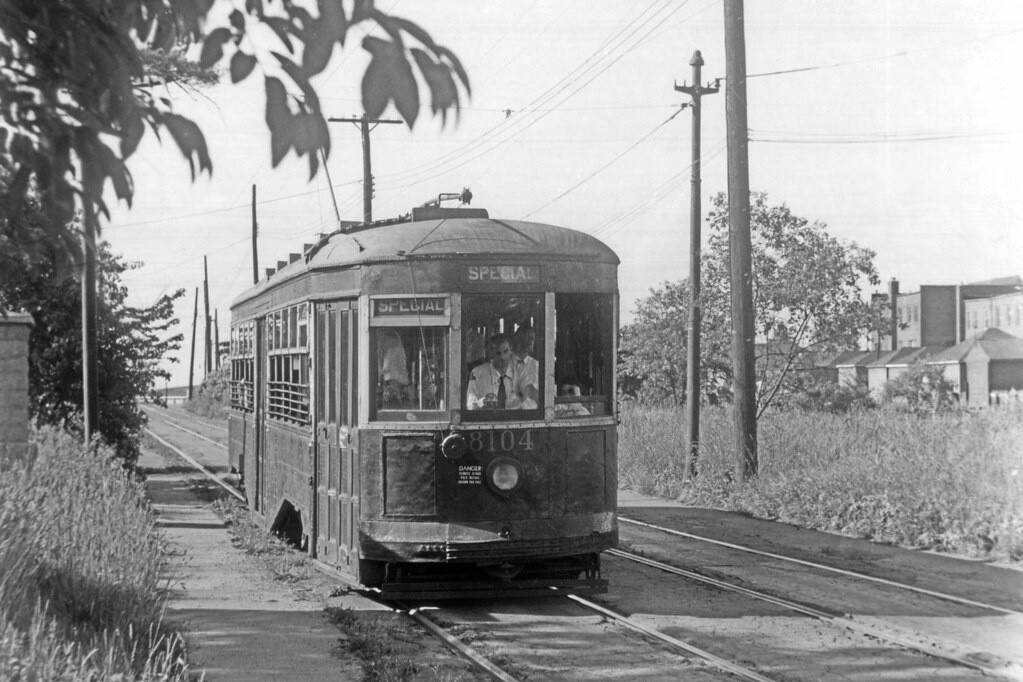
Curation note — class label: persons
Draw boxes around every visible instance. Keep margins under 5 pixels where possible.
[377,328,410,410]
[463,333,539,412]
[421,358,445,410]
[554,385,590,417]
[511,326,539,409]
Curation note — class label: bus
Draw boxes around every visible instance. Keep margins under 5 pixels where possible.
[227,207,620,603]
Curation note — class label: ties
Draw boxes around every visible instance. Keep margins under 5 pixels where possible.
[497,375,507,408]
